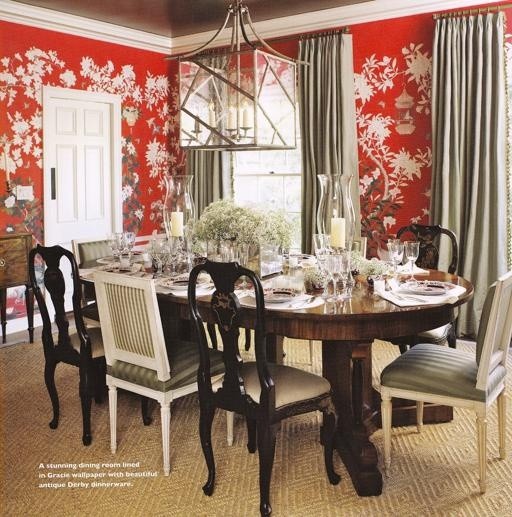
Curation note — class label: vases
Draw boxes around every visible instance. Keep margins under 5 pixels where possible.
[222,236,263,280]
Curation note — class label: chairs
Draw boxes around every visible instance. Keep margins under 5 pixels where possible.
[92,268,235,476]
[380,266,511,494]
[188,258,340,516]
[374,223,459,350]
[28,244,153,445]
[73,234,126,327]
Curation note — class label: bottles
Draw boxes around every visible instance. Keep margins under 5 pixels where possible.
[314,173,356,287]
[161,175,200,243]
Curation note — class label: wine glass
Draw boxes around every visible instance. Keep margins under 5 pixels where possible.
[100,230,280,279]
[284,236,422,307]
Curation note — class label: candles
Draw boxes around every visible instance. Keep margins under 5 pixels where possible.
[4,151,10,179]
[171,205,184,235]
[331,216,346,248]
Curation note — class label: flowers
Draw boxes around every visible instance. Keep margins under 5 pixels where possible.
[191,195,298,255]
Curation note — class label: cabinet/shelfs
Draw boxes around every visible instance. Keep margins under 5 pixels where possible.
[1,232,37,345]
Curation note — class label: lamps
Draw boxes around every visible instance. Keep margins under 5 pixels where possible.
[162,1,312,148]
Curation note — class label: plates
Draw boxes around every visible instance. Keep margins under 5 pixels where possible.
[400,279,452,296]
[96,256,118,267]
[249,288,298,305]
[160,277,207,290]
